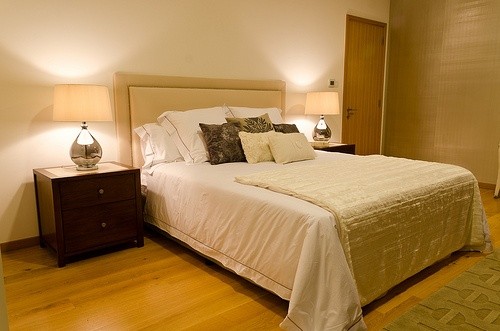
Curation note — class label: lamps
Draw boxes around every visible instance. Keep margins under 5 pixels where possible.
[52,83,114,169]
[305,91,341,142]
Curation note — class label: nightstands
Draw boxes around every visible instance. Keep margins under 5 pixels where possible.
[32,161,144,268]
[312,142,355,155]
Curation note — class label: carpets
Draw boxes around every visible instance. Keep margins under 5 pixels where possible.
[382,248,500,331]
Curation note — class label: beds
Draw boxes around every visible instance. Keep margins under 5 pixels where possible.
[114,71,493,331]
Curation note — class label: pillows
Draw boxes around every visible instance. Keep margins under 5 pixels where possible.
[270,132,315,162]
[159,106,234,164]
[199,123,248,165]
[238,131,277,163]
[228,106,284,123]
[134,123,182,165]
[225,114,274,132]
[272,123,300,133]
